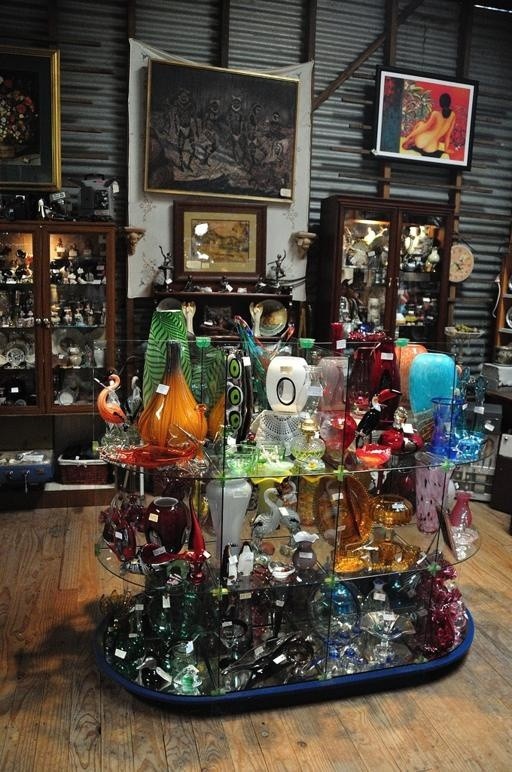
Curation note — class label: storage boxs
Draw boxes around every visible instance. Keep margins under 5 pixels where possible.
[0,448,110,485]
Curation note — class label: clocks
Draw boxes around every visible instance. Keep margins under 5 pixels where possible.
[448,245,473,282]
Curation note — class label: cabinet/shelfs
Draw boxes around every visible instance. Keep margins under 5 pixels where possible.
[319,197,451,344]
[0,217,117,413]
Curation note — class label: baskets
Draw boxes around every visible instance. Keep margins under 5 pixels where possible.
[56,443,115,486]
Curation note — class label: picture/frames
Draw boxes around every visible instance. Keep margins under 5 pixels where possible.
[141,57,302,205]
[169,198,267,280]
[367,63,479,173]
[0,40,66,193]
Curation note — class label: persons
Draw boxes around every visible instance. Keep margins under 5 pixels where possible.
[402,92,457,153]
[151,72,290,195]
[185,303,195,335]
[253,303,261,337]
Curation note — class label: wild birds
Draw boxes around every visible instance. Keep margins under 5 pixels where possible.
[125,375,143,423]
[276,481,303,546]
[249,486,284,543]
[97,374,126,433]
